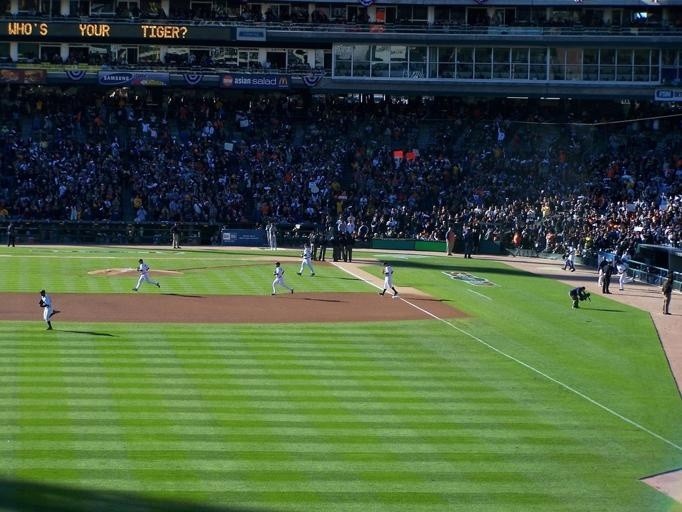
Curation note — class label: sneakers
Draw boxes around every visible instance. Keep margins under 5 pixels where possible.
[297,273,301,275]
[53,309,60,313]
[310,273,315,277]
[156,282,160,288]
[132,288,138,291]
[379,293,384,296]
[311,258,352,262]
[272,293,277,295]
[46,327,52,330]
[394,292,398,296]
[291,288,293,294]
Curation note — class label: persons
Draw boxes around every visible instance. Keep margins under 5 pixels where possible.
[601,261,613,294]
[660,273,674,315]
[378,262,398,296]
[569,287,588,308]
[0,2,682,291]
[37,289,62,331]
[270,261,293,296]
